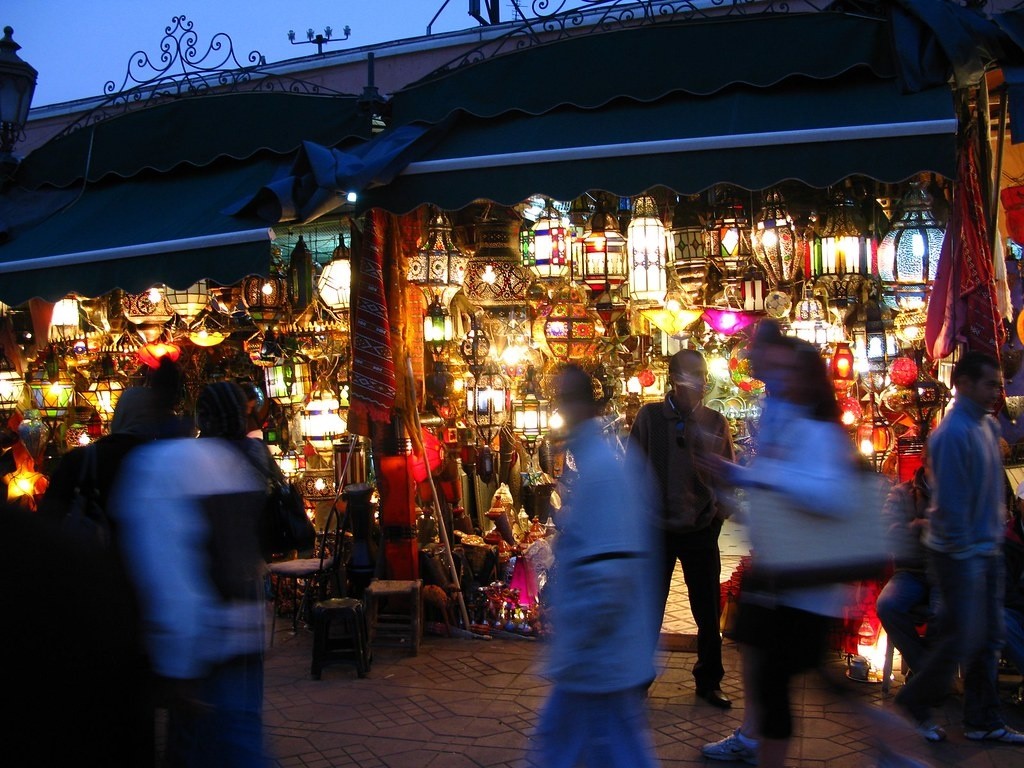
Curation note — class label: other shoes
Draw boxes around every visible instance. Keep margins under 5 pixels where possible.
[962,723,1024,745]
[694,686,732,710]
[761,720,792,739]
[919,715,951,743]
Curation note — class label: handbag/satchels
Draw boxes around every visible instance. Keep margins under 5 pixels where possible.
[746,474,898,592]
[201,485,319,599]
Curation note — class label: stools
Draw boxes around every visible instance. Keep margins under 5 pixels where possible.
[366,576,424,655]
[312,596,372,679]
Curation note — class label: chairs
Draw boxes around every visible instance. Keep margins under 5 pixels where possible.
[266,493,347,648]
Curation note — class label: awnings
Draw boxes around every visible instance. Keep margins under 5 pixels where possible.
[223,7,969,224]
[1,91,346,308]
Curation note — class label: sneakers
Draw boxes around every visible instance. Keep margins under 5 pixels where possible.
[700,727,762,766]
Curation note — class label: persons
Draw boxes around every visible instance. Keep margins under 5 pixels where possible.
[876,449,1015,703]
[524,364,660,768]
[627,349,734,710]
[0,353,283,768]
[696,317,863,764]
[894,351,1024,744]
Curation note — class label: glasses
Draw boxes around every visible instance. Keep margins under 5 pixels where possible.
[676,419,687,450]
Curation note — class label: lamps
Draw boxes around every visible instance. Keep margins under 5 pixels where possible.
[0,171,955,524]
